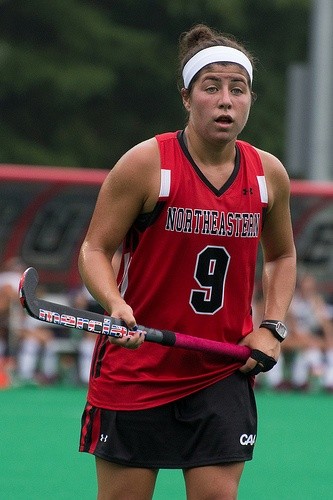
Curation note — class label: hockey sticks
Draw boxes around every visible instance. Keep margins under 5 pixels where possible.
[18,267,252,362]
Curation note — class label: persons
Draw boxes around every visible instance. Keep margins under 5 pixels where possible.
[77,26,297,499]
[248,270,333,392]
[0,254,108,388]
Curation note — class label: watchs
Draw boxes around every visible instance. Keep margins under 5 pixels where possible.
[260,318,288,343]
